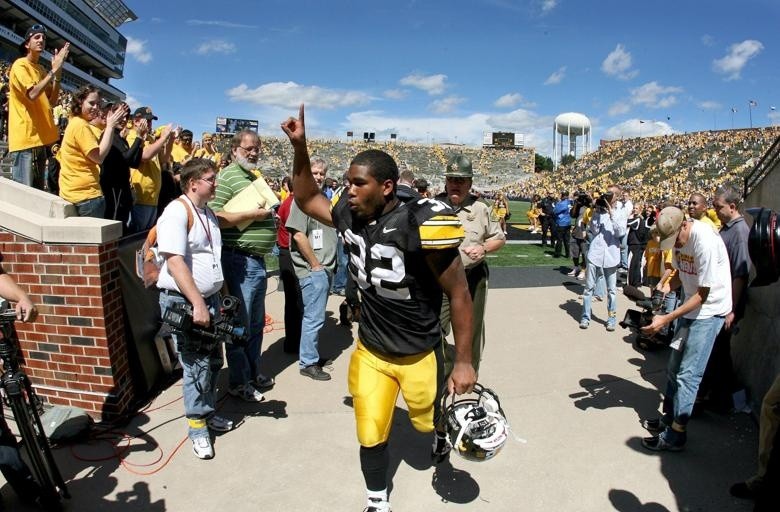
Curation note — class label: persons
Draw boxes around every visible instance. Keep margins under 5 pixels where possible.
[156,157,235,460]
[529,126,780,460]
[212,132,537,381]
[0,24,225,360]
[725,378,779,499]
[275,102,474,512]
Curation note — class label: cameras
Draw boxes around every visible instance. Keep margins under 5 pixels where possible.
[745,206,780,288]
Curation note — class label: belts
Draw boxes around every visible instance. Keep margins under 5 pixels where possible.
[221,246,264,262]
[159,288,185,298]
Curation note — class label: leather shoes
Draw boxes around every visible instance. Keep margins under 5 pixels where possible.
[300,364,331,381]
[316,357,332,366]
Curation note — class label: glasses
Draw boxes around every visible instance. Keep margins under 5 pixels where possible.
[25,24,48,39]
[197,177,216,184]
[238,145,260,153]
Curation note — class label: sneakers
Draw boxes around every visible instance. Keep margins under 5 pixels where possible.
[191,435,214,459]
[227,382,264,402]
[248,373,275,387]
[283,339,300,355]
[617,266,629,273]
[642,414,674,431]
[527,225,586,280]
[641,426,686,453]
[361,497,389,512]
[605,317,616,331]
[207,415,233,432]
[578,317,590,329]
[430,431,452,462]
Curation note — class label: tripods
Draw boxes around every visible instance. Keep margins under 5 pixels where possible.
[1,369,72,510]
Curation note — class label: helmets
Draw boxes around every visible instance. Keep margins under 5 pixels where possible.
[442,395,509,463]
[442,155,473,178]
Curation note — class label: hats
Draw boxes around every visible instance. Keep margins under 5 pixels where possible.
[655,206,684,251]
[99,96,115,109]
[560,190,570,196]
[133,106,158,121]
[19,24,48,53]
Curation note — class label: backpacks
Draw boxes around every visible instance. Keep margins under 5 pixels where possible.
[135,196,194,288]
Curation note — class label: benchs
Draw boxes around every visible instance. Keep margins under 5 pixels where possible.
[0,141,14,180]
